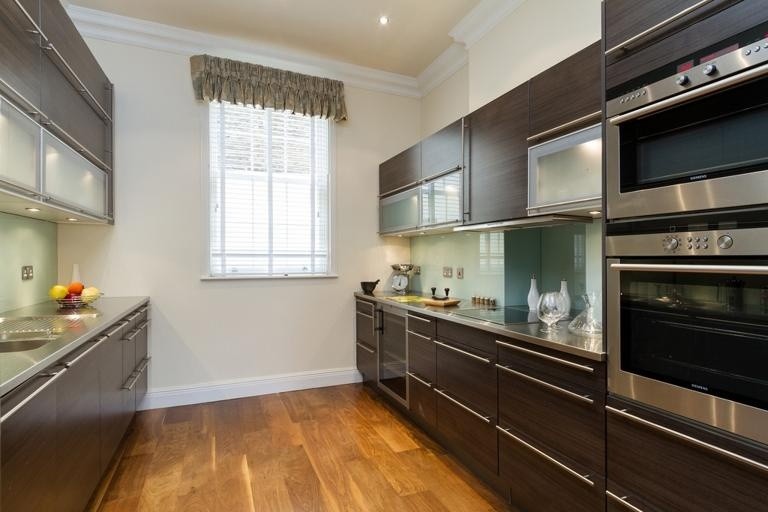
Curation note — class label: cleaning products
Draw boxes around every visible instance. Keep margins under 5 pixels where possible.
[527,274,540,313]
[528,311,539,332]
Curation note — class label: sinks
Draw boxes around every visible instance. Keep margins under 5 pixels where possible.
[0,338,56,352]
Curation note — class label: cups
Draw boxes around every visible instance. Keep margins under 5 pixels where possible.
[360,282,377,293]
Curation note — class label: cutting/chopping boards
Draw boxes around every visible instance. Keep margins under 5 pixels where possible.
[422,299,460,308]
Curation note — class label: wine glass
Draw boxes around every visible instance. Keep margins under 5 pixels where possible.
[535,292,568,333]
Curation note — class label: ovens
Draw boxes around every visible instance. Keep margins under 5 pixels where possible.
[603,36,767,447]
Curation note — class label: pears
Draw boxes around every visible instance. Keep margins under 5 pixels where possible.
[80,287,104,304]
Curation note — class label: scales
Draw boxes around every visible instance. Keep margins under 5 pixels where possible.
[390,264,413,296]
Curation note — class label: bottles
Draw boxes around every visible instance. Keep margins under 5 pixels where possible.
[568,294,602,338]
[70,263,82,284]
[526,272,539,311]
[471,296,496,306]
[527,311,541,336]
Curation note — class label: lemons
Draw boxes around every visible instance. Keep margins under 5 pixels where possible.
[48,286,68,299]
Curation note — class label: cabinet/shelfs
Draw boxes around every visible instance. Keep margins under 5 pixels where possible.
[60,336,104,512]
[354,293,379,392]
[120,309,141,441]
[434,316,496,489]
[136,302,153,409]
[604,389,767,511]
[524,39,602,221]
[0,346,69,512]
[460,78,529,230]
[40,0,117,226]
[97,321,128,470]
[0,1,39,221]
[598,0,766,102]
[496,331,605,512]
[406,309,434,438]
[377,110,461,240]
